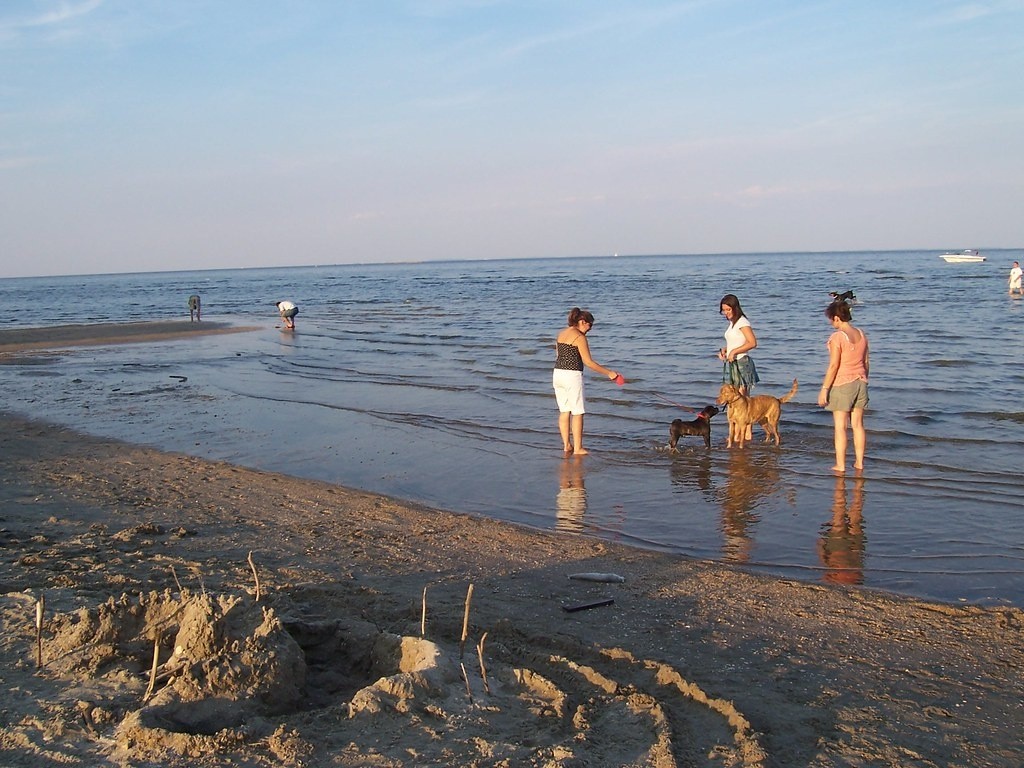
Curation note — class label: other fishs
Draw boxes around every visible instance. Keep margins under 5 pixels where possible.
[568,572,625,584]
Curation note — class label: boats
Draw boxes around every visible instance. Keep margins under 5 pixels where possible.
[938,248,986,265]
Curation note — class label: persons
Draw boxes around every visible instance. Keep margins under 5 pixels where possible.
[553,307,621,455]
[276,301,299,328]
[1008,261,1024,295]
[718,294,760,441]
[818,301,869,471]
[188,295,201,322]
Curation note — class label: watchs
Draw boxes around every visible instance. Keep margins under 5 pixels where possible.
[822,386,829,390]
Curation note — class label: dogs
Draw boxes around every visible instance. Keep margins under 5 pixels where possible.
[716,378,798,450]
[669,405,719,448]
